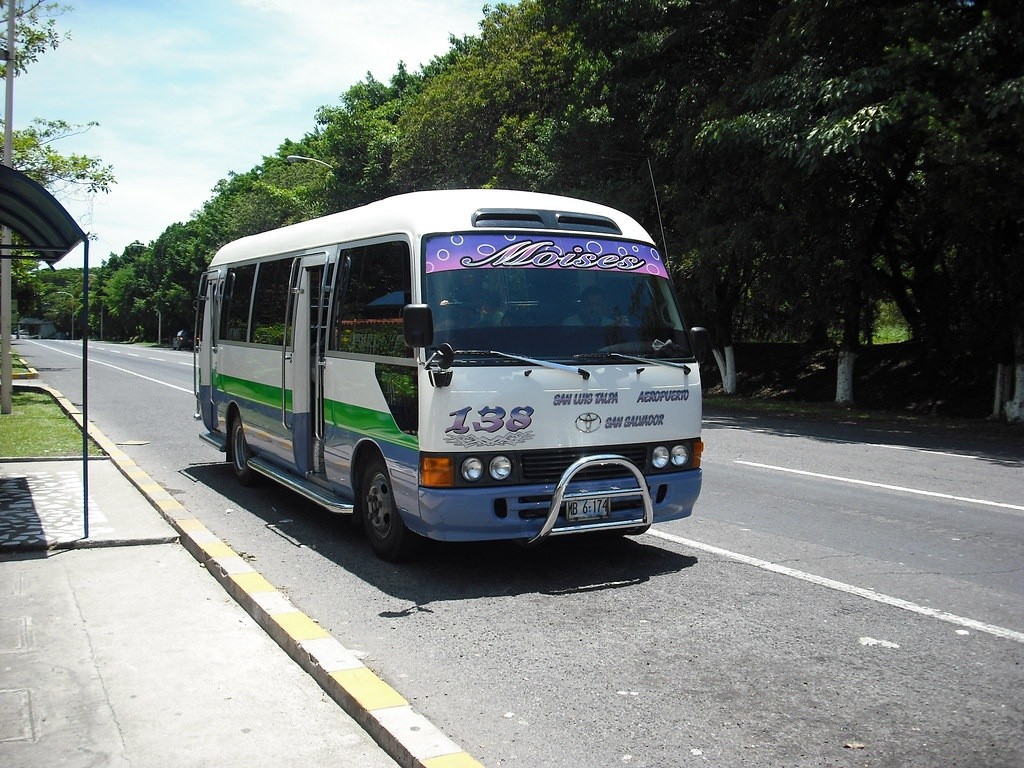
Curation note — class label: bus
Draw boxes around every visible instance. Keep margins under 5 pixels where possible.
[193,189,709,565]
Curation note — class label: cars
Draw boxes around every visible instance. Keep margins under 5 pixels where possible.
[173,329,199,351]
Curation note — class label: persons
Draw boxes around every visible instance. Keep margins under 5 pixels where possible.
[561,287,633,354]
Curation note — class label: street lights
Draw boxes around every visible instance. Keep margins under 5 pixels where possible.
[58,291,74,340]
